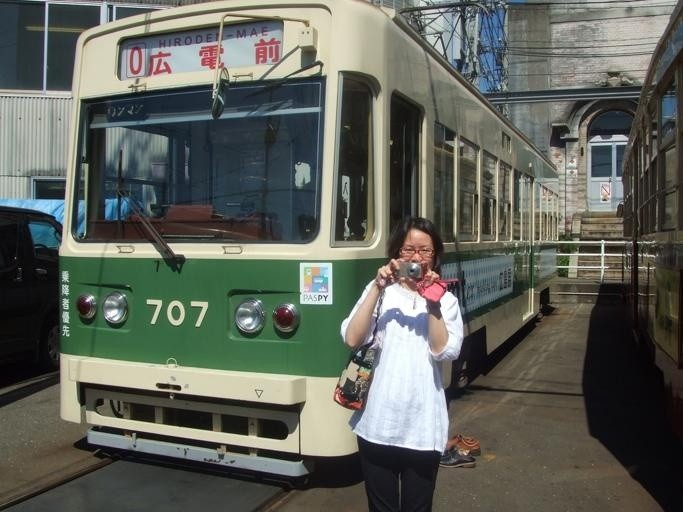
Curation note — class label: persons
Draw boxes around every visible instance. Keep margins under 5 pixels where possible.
[332,214,463,510]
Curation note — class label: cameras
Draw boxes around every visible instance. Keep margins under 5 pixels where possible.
[394,261,423,280]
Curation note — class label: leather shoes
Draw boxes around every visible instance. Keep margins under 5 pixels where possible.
[439,434,480,467]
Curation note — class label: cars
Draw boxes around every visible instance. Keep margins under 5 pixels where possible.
[0,205,63,375]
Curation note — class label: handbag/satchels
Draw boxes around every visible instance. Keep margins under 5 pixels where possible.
[334,338,378,410]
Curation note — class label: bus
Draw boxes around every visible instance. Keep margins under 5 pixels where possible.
[53,0,560,481]
[619,3,679,409]
